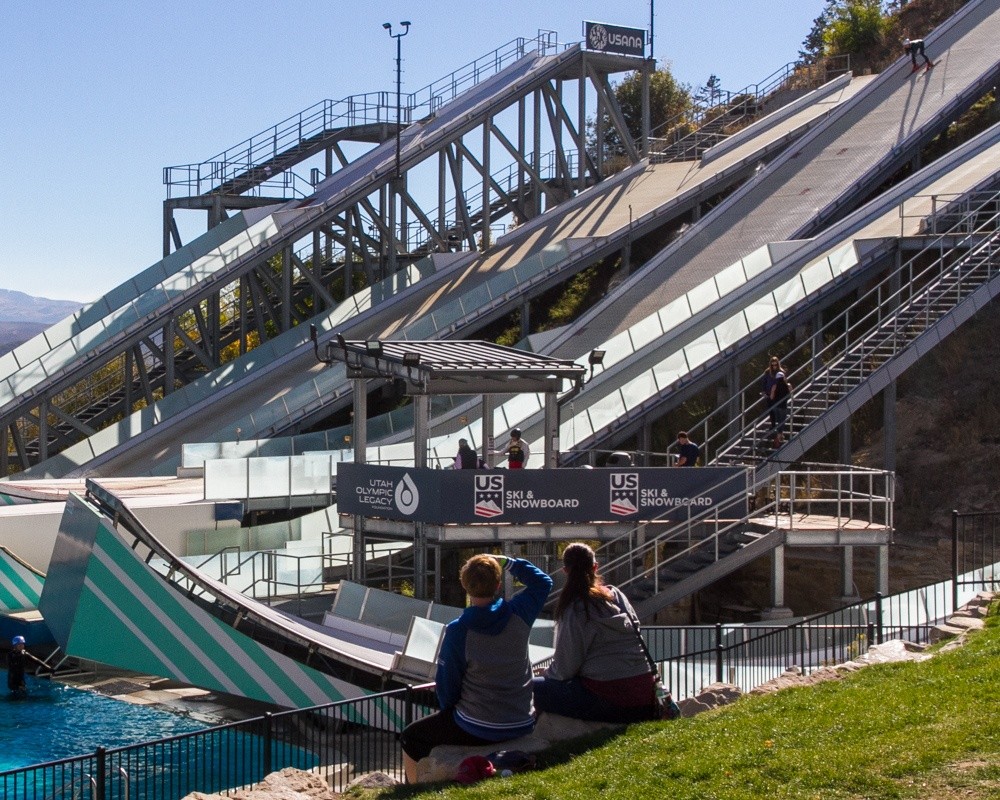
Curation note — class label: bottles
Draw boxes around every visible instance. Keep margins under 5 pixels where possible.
[656,681,670,708]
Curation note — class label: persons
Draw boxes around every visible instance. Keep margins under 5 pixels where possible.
[401,553,554,785]
[7,636,25,696]
[760,357,792,447]
[534,542,659,723]
[673,432,698,467]
[454,439,479,470]
[903,39,934,72]
[498,428,530,469]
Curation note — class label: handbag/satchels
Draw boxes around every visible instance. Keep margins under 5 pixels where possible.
[650,671,681,719]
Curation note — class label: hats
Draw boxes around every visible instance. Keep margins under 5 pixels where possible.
[775,371,784,379]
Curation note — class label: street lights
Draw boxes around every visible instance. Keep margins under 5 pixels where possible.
[381,19,414,180]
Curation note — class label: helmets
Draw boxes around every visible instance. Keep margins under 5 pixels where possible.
[510,428,521,440]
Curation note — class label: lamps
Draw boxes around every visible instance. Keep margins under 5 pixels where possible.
[582,351,605,385]
[336,332,362,371]
[403,353,424,388]
[365,339,384,374]
[310,323,333,364]
